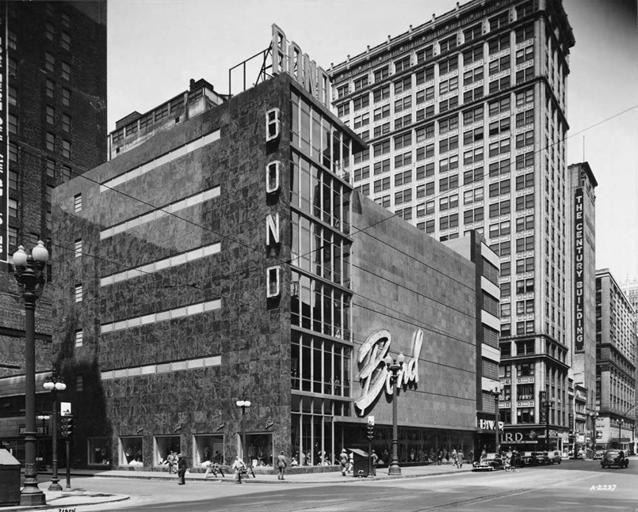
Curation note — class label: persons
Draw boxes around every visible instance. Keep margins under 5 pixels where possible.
[370,450,378,476]
[166,449,354,485]
[437,447,522,472]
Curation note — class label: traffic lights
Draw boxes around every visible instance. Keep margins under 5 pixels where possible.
[65,416,74,440]
[59,414,65,440]
[371,427,375,440]
[366,427,370,440]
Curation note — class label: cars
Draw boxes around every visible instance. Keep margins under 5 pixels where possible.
[470,446,630,472]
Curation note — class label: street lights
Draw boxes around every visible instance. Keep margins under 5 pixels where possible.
[540,396,556,448]
[237,385,250,470]
[42,371,66,492]
[588,410,600,452]
[616,418,624,449]
[378,350,407,476]
[488,381,505,453]
[12,240,49,505]
